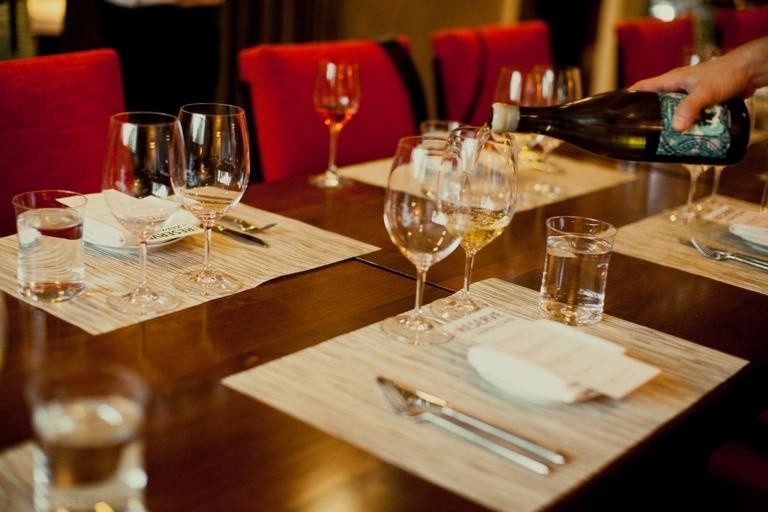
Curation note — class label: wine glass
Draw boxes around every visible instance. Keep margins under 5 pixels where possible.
[432,127,518,323]
[169,103,251,297]
[306,61,361,190]
[496,67,582,204]
[102,111,187,317]
[663,164,727,225]
[379,136,471,346]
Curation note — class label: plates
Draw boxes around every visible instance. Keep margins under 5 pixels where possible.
[465,319,626,402]
[73,198,201,254]
[728,224,768,251]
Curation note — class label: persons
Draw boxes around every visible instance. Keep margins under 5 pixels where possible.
[627,34,768,132]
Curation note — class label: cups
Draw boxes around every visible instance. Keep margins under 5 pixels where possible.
[537,216,616,326]
[684,44,721,67]
[27,360,152,512]
[12,190,87,303]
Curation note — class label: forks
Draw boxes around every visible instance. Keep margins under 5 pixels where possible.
[377,377,549,476]
[691,237,768,272]
[222,212,277,233]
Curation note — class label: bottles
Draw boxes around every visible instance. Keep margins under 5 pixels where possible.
[488,90,750,166]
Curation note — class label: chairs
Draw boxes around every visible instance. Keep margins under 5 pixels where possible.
[238,35,431,182]
[715,7,768,44]
[428,21,554,133]
[616,12,698,89]
[0,48,135,236]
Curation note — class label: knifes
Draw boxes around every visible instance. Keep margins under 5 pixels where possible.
[391,380,565,465]
[210,222,269,252]
[678,237,768,268]
